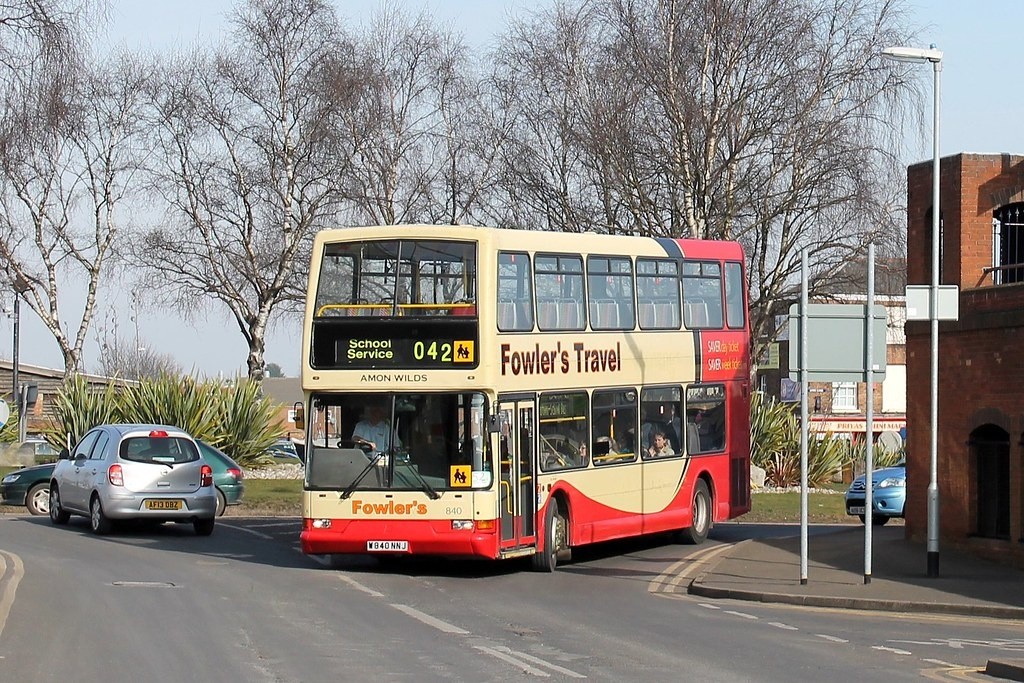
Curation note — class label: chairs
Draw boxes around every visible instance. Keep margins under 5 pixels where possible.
[345,298,735,331]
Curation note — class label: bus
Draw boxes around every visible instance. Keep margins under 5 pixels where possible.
[292,223,754,572]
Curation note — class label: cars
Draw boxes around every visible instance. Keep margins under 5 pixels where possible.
[49,423,217,536]
[845,456,907,525]
[266,441,295,457]
[0,436,245,515]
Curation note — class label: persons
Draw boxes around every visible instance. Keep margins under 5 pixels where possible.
[351,409,402,459]
[597,436,622,462]
[648,430,674,457]
[578,439,586,457]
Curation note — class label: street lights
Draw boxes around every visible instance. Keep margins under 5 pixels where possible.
[11,274,25,409]
[883,47,944,578]
